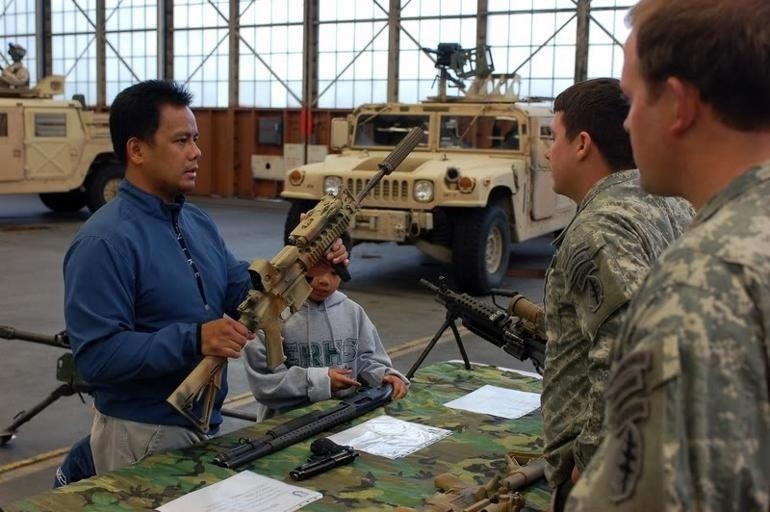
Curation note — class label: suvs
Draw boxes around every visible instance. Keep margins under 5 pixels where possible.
[280,44,579,298]
[0,74,127,214]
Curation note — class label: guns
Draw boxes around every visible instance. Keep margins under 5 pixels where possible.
[213,382,393,468]
[406,275,548,379]
[289,437,360,481]
[424,449,544,512]
[165,126,425,434]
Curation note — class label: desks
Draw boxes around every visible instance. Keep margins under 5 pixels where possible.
[0,356,555,511]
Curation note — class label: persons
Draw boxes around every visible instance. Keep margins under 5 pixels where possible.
[239,198,412,425]
[558,1,770,512]
[60,77,350,480]
[538,77,697,512]
[1,44,30,88]
